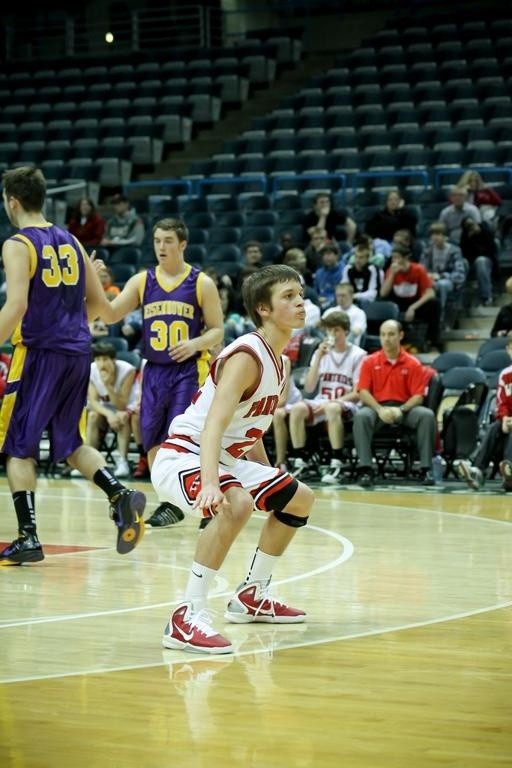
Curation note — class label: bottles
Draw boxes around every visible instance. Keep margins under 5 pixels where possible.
[326,333,336,356]
[430,453,444,484]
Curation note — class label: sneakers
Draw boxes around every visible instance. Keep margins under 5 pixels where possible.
[452,459,484,490]
[274,457,373,487]
[224,579,308,624]
[53,465,84,479]
[144,502,185,529]
[0,528,44,566]
[133,457,149,477]
[497,460,512,492]
[109,487,146,554]
[162,601,235,654]
[418,468,435,485]
[114,461,130,478]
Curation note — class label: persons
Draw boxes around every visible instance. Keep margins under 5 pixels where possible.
[0,164,146,568]
[150,265,312,656]
[95,215,228,529]
[2,167,512,492]
